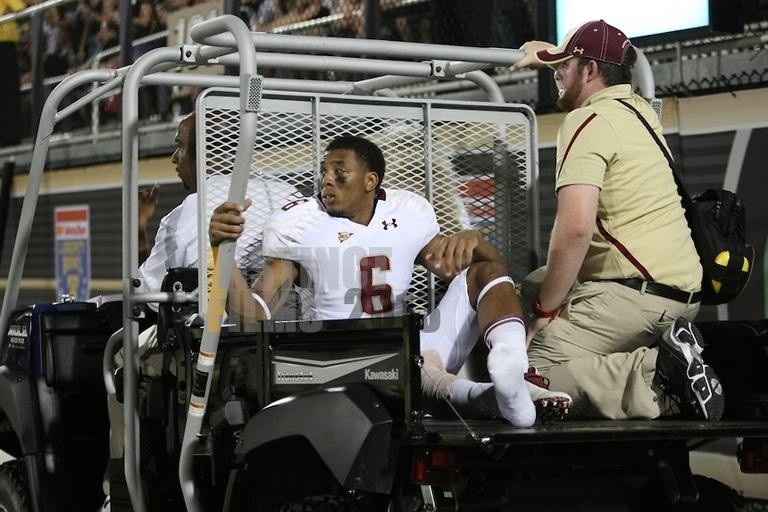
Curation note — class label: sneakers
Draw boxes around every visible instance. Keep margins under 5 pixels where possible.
[522,366,572,421]
[652,317,726,423]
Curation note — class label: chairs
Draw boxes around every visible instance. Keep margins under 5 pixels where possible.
[115,268,198,404]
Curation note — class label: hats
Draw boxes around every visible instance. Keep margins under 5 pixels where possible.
[536,20,635,69]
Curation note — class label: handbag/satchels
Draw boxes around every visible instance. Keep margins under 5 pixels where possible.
[688,188,755,307]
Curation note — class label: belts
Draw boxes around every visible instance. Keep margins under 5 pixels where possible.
[614,278,701,306]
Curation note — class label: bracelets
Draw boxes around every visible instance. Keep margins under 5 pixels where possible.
[532,295,560,319]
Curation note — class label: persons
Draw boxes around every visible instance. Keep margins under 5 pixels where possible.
[207,131,575,429]
[508,17,729,421]
[96,108,305,510]
[0,1,535,146]
